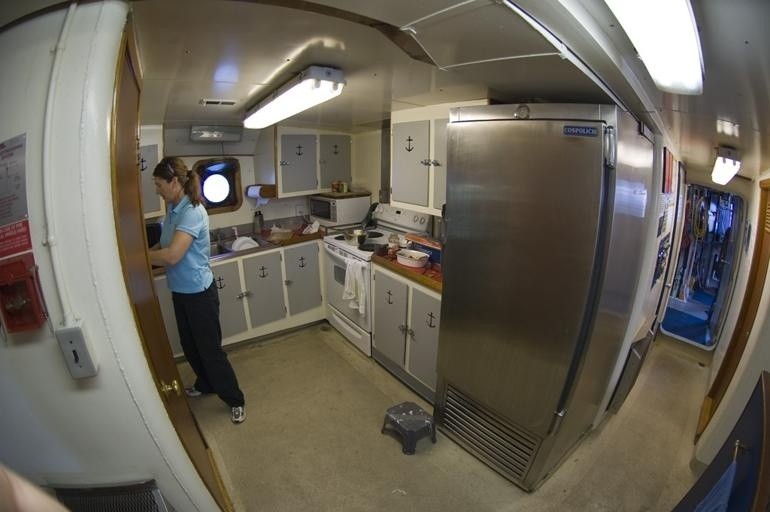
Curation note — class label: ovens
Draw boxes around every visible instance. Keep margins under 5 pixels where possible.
[323,241,371,334]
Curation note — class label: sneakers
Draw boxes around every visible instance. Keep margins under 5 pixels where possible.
[185,387,202,397]
[231,406,246,423]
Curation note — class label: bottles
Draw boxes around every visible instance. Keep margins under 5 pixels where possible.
[254,211,264,234]
[331,181,349,193]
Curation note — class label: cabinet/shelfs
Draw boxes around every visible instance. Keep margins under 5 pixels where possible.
[139,125,166,220]
[372,269,441,407]
[255,125,352,201]
[390,99,489,218]
[153,240,322,363]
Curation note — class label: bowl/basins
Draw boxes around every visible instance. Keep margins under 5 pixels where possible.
[395,248,430,268]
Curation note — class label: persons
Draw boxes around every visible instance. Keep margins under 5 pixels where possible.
[145,154,249,427]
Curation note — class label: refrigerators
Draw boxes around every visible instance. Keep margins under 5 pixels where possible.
[431,104,656,494]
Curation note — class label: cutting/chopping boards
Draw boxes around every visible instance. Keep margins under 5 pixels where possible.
[266,232,295,245]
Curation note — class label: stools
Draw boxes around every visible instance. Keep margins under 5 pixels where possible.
[382,402,437,455]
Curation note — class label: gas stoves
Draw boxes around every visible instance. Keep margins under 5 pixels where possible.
[323,226,408,262]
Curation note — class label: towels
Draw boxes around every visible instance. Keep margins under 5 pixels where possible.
[341,258,366,313]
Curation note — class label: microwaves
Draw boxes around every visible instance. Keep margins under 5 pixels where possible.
[308,196,371,228]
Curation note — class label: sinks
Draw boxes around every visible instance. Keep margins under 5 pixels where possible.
[223,236,269,251]
[210,241,232,260]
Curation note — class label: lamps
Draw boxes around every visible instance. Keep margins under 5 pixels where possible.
[711,146,742,186]
[242,66,348,130]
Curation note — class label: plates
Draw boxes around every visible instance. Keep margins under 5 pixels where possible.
[231,236,259,251]
